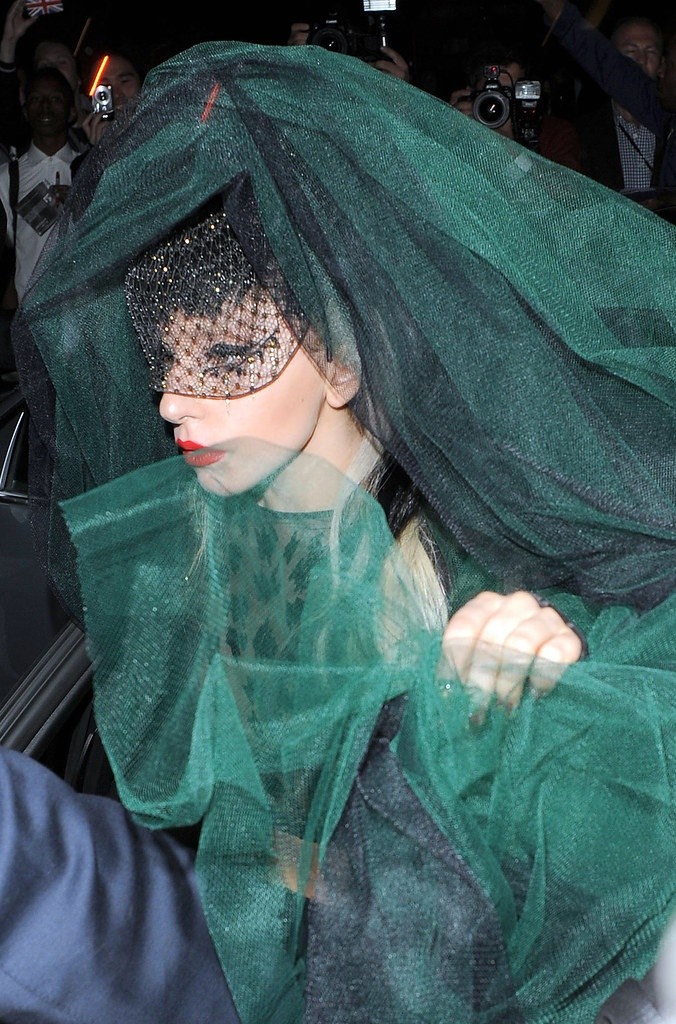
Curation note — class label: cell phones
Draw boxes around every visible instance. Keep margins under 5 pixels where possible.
[25,0,64,17]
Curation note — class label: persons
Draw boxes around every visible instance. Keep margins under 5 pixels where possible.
[0,0,676,1024]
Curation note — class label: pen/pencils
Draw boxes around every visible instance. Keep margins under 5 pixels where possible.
[55,171,60,208]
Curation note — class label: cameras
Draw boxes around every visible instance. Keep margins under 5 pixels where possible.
[463,63,547,152]
[307,0,397,66]
[92,84,115,123]
